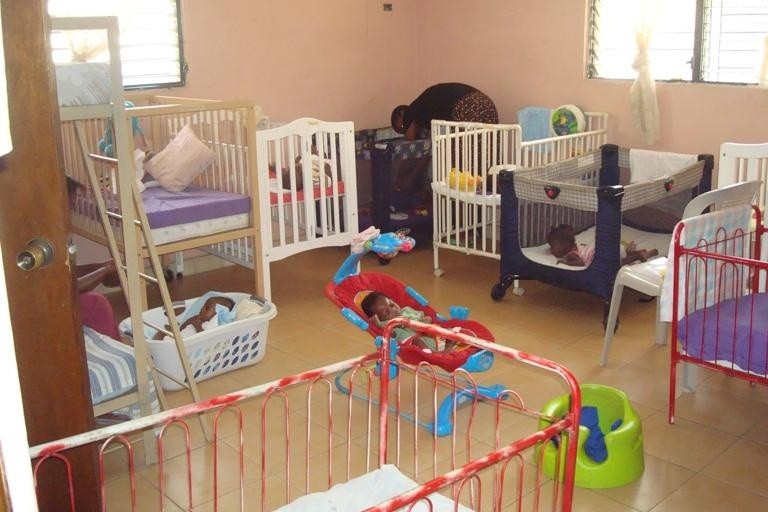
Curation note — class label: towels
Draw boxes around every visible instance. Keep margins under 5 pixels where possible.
[628,148,698,218]
[659,204,754,323]
[516,106,553,154]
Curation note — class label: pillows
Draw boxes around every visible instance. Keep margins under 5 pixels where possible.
[177,291,252,327]
[145,122,216,193]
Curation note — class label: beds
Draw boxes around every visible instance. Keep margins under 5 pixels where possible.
[489,143,715,335]
[45,15,213,466]
[165,107,360,303]
[62,93,265,311]
[428,107,611,298]
[30,314,584,512]
[355,124,434,264]
[666,205,768,424]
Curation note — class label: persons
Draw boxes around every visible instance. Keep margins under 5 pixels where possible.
[546,222,659,267]
[362,290,461,353]
[390,82,500,245]
[152,294,234,343]
[268,143,332,192]
[63,173,127,345]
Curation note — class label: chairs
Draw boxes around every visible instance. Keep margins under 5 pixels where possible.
[714,142,768,297]
[596,181,763,395]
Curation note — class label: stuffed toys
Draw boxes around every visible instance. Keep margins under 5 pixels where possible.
[98,99,146,159]
[445,167,482,193]
